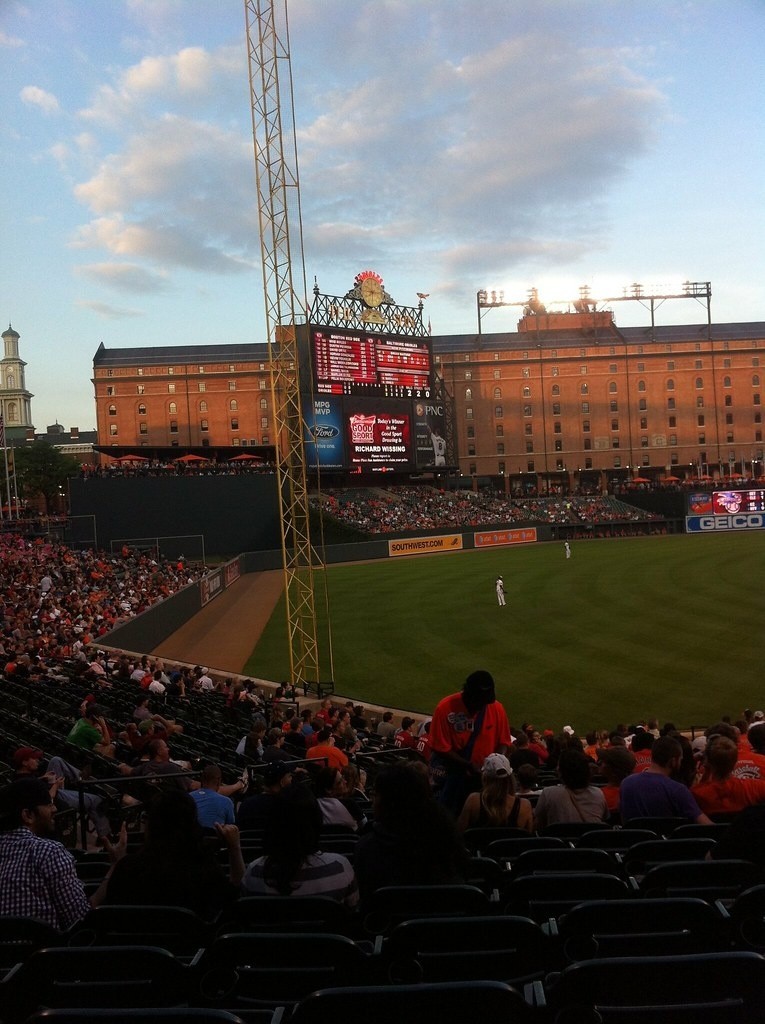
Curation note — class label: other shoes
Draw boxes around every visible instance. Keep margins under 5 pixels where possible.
[238,768,250,794]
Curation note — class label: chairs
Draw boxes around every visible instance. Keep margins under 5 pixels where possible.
[0,674,765,1024]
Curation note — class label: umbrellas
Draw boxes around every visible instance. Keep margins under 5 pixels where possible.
[228,454,262,460]
[114,455,146,461]
[174,454,209,461]
[665,476,678,480]
[633,477,650,482]
[702,474,742,479]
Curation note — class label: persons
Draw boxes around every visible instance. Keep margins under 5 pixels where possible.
[0,532,765,1024]
[81,460,277,478]
[0,511,69,530]
[301,475,765,538]
[496,575,507,607]
[564,540,571,559]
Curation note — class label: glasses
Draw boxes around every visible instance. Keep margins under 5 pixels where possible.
[338,774,345,784]
[38,801,53,806]
[597,759,602,766]
[369,790,382,798]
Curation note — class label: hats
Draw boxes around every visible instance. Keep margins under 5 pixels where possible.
[481,752,513,778]
[597,746,636,769]
[465,670,496,704]
[563,725,574,735]
[13,747,44,762]
